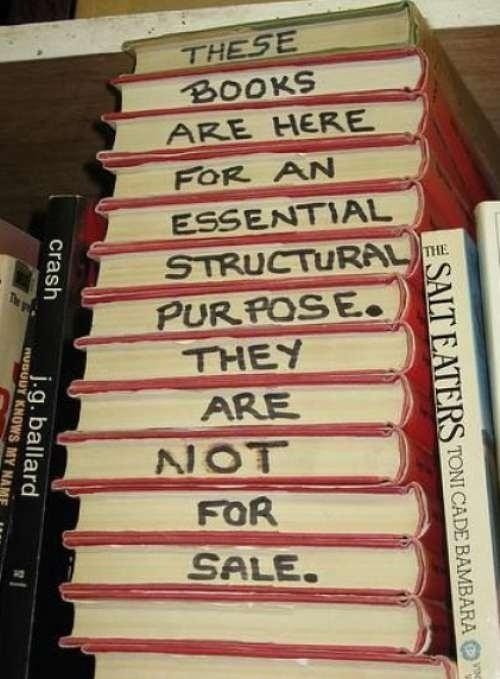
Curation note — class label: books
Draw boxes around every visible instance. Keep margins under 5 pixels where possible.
[0,1,500,679]
